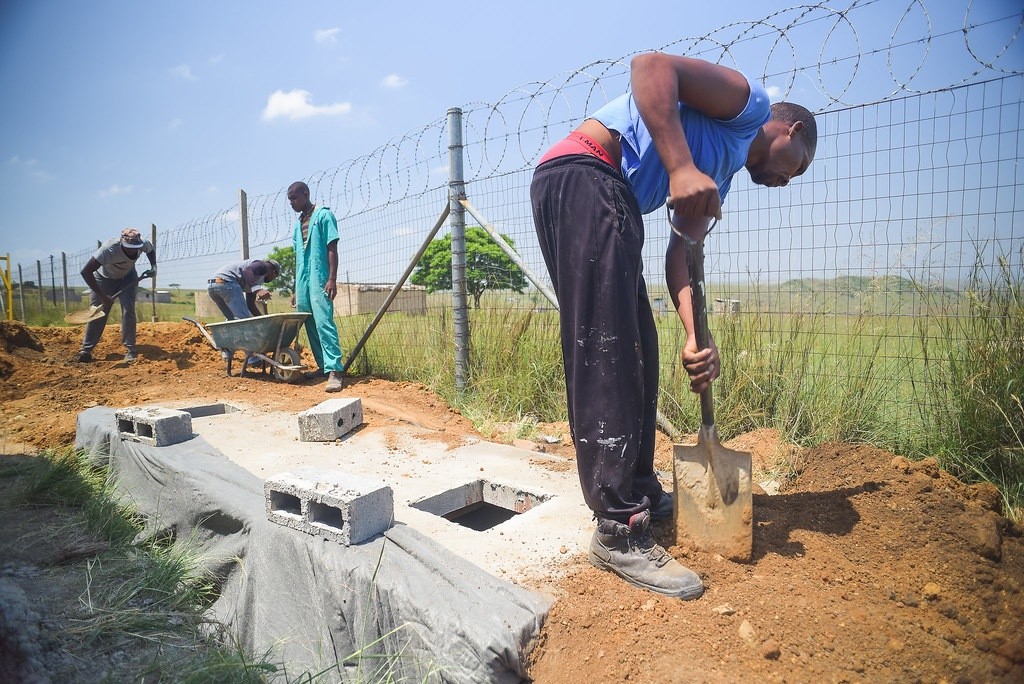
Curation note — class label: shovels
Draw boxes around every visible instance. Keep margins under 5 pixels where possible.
[255,292,272,315]
[63,270,155,324]
[665,195,753,563]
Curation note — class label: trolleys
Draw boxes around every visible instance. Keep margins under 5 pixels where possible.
[182,311,314,384]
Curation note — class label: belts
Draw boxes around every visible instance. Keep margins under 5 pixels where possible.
[207,279,232,284]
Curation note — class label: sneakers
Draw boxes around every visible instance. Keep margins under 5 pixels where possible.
[588,511,704,602]
[647,490,675,522]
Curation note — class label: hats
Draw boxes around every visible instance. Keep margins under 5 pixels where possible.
[266,259,280,277]
[120,228,144,248]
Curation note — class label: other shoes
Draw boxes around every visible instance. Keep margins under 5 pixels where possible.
[250,361,264,367]
[325,371,344,392]
[222,353,228,362]
[74,353,92,363]
[124,344,137,365]
[304,368,328,379]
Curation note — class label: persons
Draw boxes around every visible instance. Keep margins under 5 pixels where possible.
[75,228,157,362]
[287,181,344,393]
[531,53,817,601]
[207,259,280,367]
